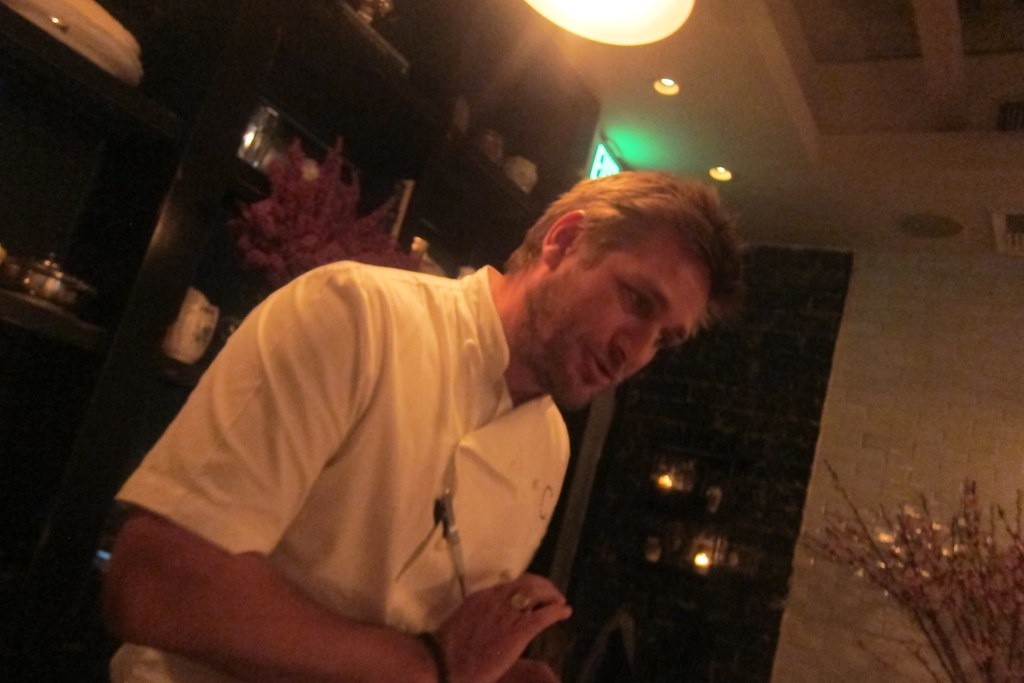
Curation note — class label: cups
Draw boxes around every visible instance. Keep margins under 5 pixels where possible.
[159,285,222,367]
[504,153,539,196]
[476,126,507,165]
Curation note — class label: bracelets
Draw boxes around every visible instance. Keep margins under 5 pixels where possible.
[419,630,451,683]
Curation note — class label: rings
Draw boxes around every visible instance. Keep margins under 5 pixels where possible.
[507,590,531,612]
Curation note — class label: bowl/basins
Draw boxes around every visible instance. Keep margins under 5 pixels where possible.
[23,258,87,310]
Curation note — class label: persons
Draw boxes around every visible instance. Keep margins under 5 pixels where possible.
[103,172,745,683]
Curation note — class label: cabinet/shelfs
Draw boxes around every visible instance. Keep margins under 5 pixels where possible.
[0,0,601,624]
